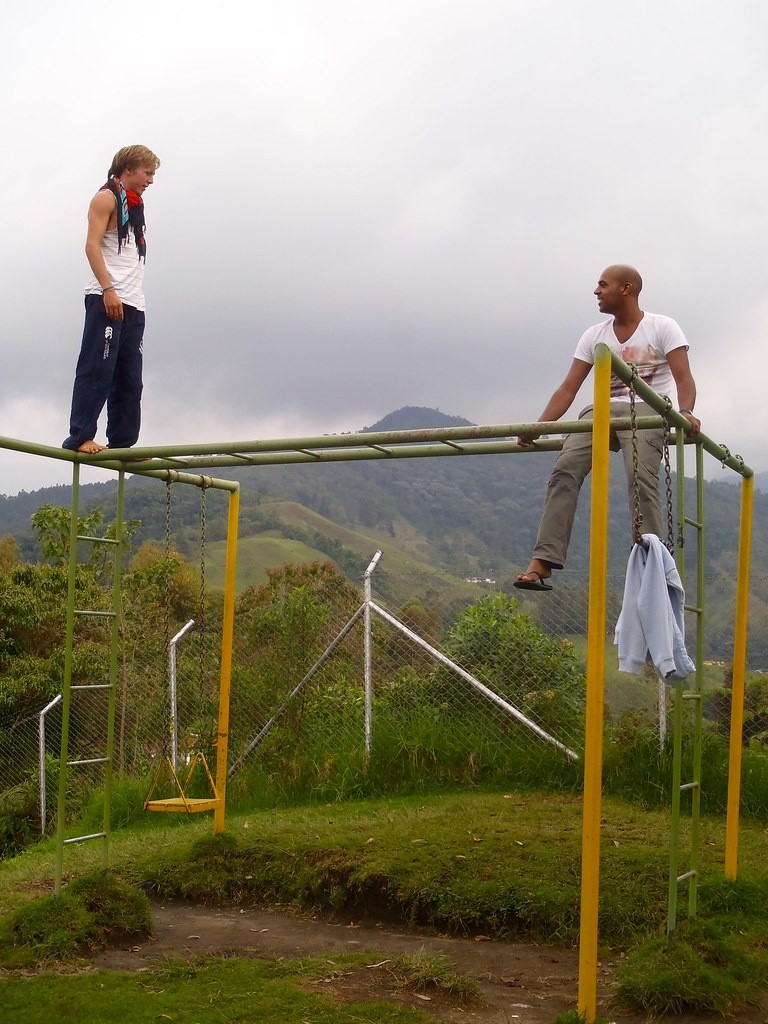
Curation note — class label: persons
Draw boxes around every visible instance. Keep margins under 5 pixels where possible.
[63,143,159,455]
[512,264,700,592]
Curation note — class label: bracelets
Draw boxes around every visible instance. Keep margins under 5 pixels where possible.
[101,286,115,292]
[679,409,693,415]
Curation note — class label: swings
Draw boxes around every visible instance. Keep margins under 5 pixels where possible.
[142,478,227,811]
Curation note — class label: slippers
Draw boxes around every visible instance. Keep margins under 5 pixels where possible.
[513,571,552,591]
